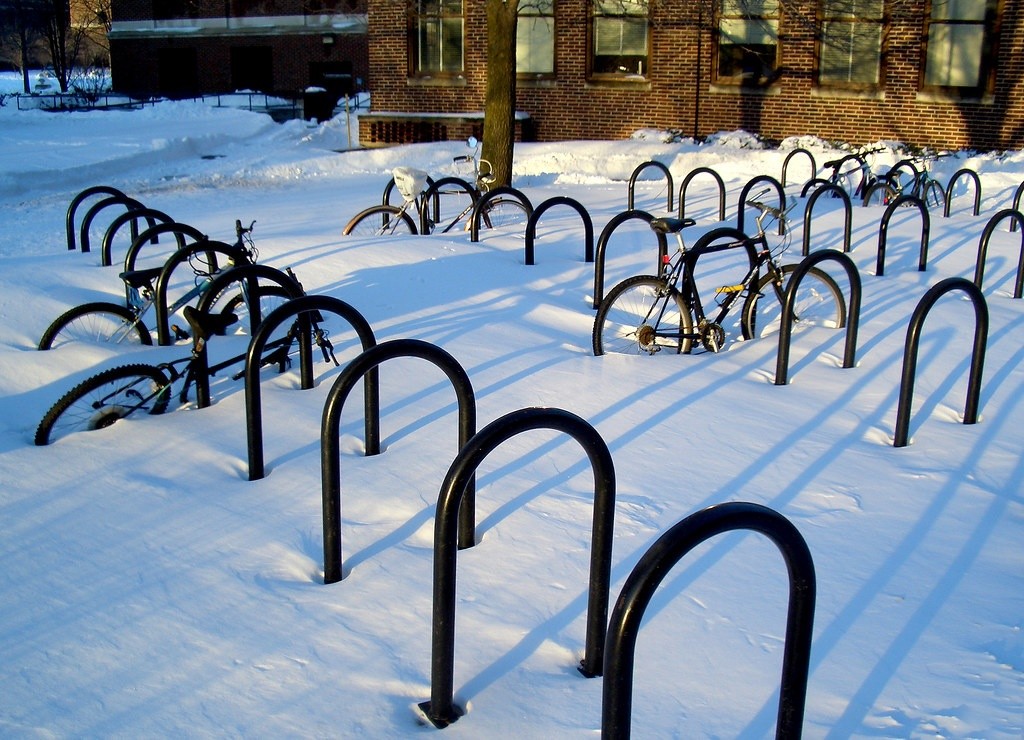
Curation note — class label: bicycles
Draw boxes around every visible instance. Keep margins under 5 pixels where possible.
[37,217,260,354]
[32,267,340,448]
[800,147,888,200]
[341,138,531,238]
[591,187,848,357]
[862,148,951,212]
